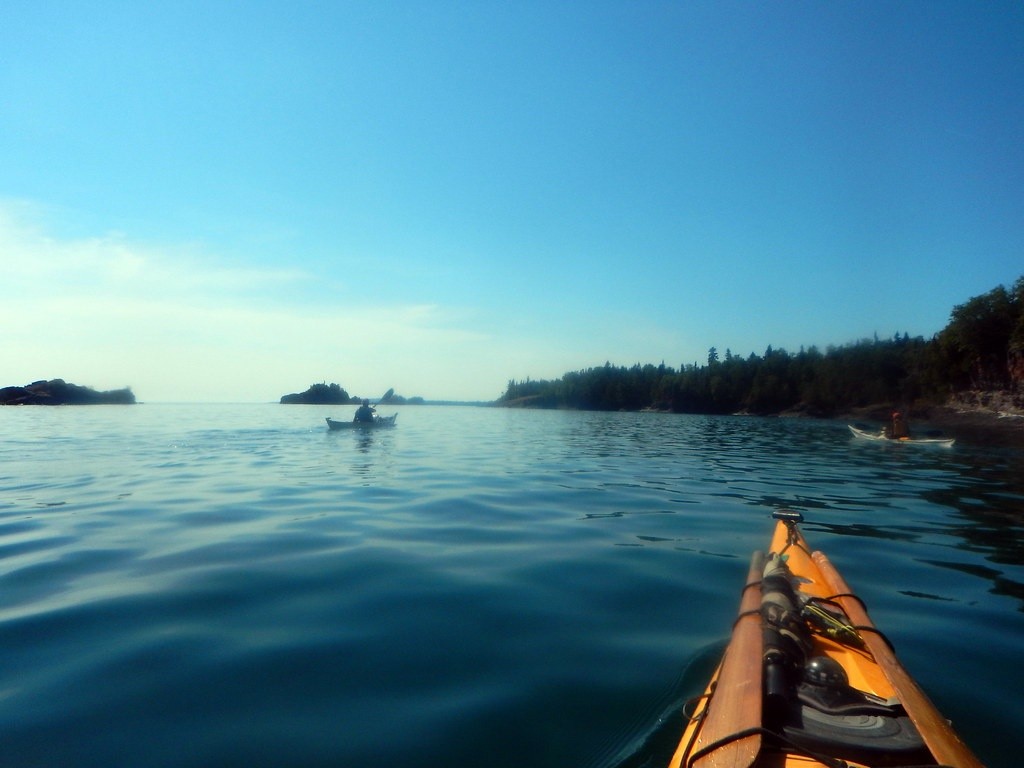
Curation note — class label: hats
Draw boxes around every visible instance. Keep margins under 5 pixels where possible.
[893,412,902,418]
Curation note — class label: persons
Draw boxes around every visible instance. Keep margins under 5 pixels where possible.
[353,399,376,422]
[893,413,910,437]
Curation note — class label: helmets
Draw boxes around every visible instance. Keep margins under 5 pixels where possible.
[804,656,849,705]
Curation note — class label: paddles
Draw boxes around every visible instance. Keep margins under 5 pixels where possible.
[686,552,764,768]
[357,387,393,420]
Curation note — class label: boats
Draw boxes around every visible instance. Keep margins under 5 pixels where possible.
[847,424,957,448]
[325,412,398,429]
